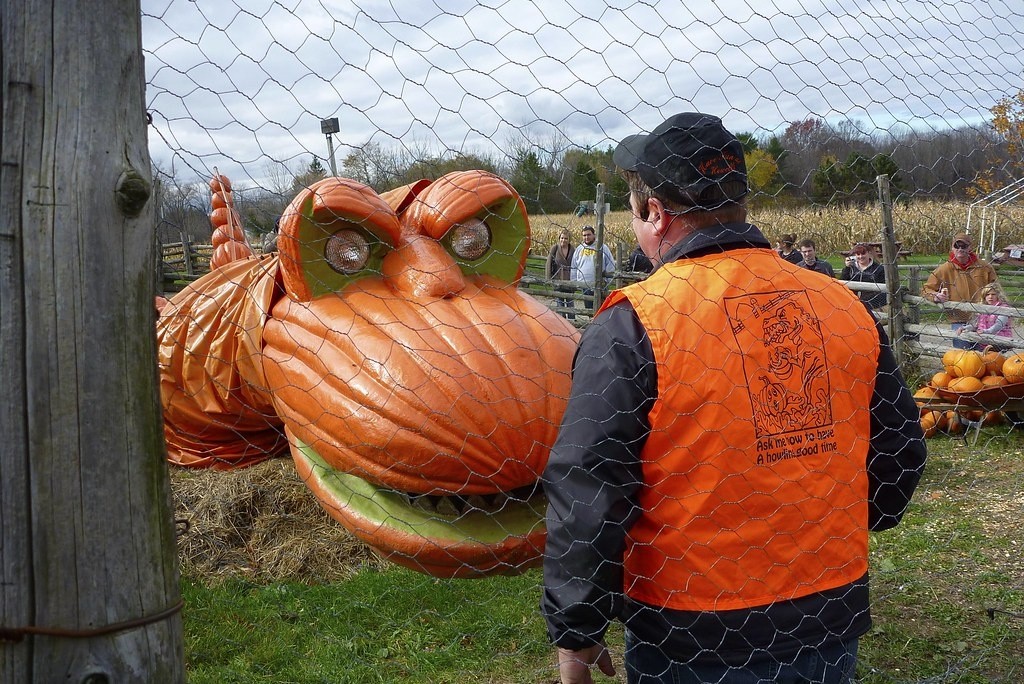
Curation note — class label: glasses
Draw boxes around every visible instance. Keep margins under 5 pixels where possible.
[582,226,591,231]
[953,243,969,249]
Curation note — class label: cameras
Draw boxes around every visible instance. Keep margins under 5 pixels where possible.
[849,256,857,263]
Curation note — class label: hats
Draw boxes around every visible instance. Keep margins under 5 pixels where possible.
[951,233,972,247]
[614,112,750,207]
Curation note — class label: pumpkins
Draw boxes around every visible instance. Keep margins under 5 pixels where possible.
[912,344,1024,438]
[209,174,251,271]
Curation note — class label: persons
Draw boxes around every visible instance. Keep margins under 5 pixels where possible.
[540,113,928,684]
[626,247,653,273]
[955,283,1012,351]
[921,232,998,350]
